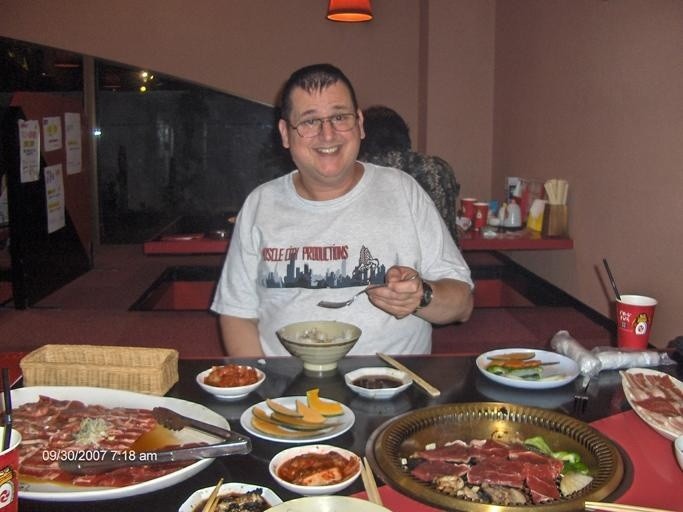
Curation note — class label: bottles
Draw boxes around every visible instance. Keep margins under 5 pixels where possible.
[497,202,506,225]
[503,200,523,227]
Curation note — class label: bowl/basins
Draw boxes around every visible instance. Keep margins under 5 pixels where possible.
[674,434,683,469]
[275,318,364,372]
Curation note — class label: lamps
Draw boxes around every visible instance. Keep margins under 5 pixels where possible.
[326,0,374,23]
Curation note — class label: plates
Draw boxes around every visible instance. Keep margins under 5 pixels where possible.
[179,482,284,512]
[622,366,683,441]
[268,443,361,495]
[264,495,397,511]
[195,365,267,399]
[239,394,356,443]
[0,385,231,502]
[342,366,412,401]
[474,347,580,390]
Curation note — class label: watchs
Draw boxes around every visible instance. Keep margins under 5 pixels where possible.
[410,276,433,316]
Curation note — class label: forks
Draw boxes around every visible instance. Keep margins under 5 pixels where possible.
[316,271,420,309]
[572,376,592,418]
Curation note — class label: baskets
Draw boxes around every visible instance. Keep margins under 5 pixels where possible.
[19,344,179,396]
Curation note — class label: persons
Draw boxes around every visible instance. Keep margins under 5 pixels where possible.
[355,103,461,251]
[209,61,476,358]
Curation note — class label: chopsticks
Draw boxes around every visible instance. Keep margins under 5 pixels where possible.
[583,499,674,512]
[358,455,383,505]
[376,350,441,400]
[203,477,225,511]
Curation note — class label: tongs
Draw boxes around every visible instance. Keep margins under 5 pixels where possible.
[58,405,253,475]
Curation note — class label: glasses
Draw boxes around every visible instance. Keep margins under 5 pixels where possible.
[290,114,359,138]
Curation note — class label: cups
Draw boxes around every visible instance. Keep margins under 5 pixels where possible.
[459,197,477,218]
[471,202,490,231]
[0,424,21,512]
[614,293,659,351]
